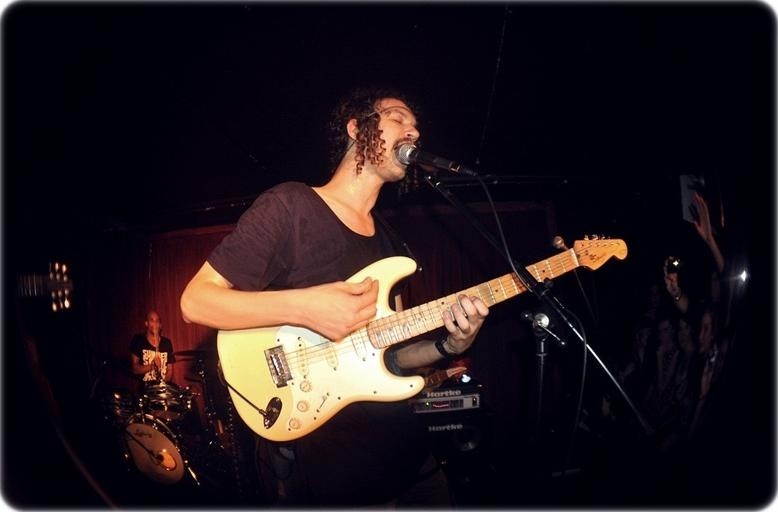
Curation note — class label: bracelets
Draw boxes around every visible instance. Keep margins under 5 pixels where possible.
[435,335,466,361]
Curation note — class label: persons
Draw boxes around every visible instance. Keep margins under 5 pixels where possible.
[128,310,176,397]
[178,85,491,510]
[628,187,721,427]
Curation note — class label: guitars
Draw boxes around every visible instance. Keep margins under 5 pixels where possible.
[215,234,629,444]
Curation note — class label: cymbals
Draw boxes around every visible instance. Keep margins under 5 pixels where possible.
[173,349,199,356]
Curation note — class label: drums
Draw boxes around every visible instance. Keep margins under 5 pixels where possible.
[124,413,188,482]
[110,391,144,419]
[144,384,190,421]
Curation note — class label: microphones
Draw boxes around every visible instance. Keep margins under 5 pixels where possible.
[397,144,481,179]
[552,235,569,252]
[535,312,550,328]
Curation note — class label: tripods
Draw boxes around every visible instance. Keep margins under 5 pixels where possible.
[190,369,235,483]
